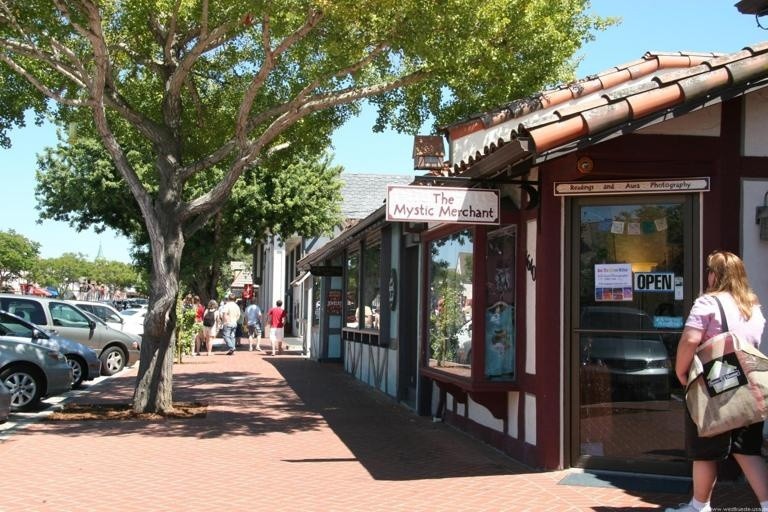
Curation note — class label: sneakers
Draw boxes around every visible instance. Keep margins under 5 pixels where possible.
[248,347,253,352]
[665,499,697,512]
[255,346,262,351]
[227,348,236,355]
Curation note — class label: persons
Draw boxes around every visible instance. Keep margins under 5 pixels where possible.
[665,250,768,512]
[181,293,286,356]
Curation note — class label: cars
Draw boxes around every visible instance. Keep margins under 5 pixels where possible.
[446,319,472,364]
[580,305,673,403]
[0,282,149,425]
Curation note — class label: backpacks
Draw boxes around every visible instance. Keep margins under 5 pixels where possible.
[203,309,215,327]
[182,304,195,326]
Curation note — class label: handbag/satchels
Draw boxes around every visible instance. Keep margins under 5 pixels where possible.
[683,294,768,439]
